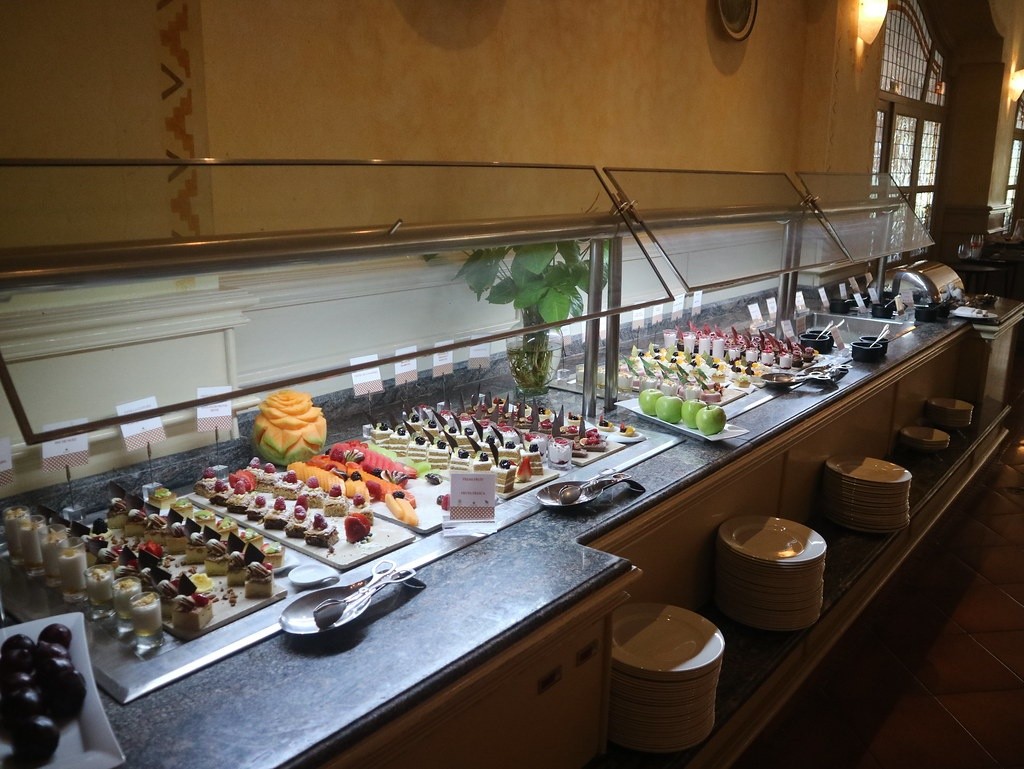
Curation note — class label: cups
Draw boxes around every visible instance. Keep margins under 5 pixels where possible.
[128,591,164,649]
[37,523,67,588]
[957,244,971,263]
[2,505,31,566]
[82,565,115,619]
[419,405,574,471]
[55,537,88,604]
[663,329,793,373]
[110,576,142,634]
[15,515,46,577]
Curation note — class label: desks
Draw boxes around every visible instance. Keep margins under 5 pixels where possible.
[943,256,1010,298]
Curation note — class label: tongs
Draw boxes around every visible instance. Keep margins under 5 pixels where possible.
[824,363,852,372]
[312,559,416,628]
[558,468,632,505]
[771,371,831,381]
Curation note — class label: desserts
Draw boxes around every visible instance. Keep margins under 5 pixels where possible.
[44,322,816,634]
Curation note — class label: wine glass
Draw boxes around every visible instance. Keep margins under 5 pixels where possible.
[970,232,984,264]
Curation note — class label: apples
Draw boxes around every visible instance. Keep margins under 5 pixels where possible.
[639,389,664,416]
[697,403,726,436]
[656,396,684,424]
[682,398,707,428]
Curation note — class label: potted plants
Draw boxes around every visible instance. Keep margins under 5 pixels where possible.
[420,234,613,395]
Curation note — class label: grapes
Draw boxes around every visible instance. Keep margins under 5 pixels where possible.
[1,623,86,763]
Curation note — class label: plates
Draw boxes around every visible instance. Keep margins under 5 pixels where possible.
[597,419,645,443]
[714,515,828,632]
[613,397,750,442]
[272,553,300,573]
[824,454,912,534]
[536,473,603,509]
[608,603,726,753]
[949,310,998,319]
[289,562,340,588]
[759,373,804,389]
[804,367,849,385]
[0,611,126,769]
[926,398,974,430]
[900,426,950,453]
[278,587,372,635]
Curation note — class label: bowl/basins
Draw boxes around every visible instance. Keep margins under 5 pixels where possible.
[850,342,883,362]
[871,304,894,319]
[799,330,835,354]
[913,306,939,322]
[828,299,853,314]
[859,336,890,355]
